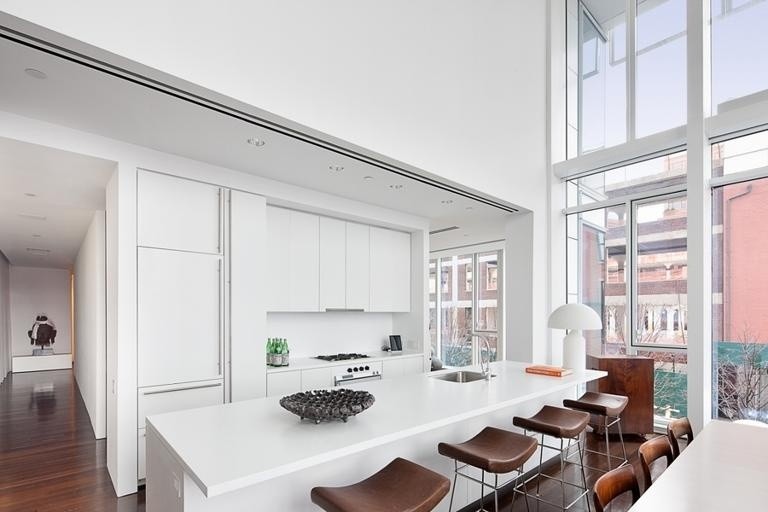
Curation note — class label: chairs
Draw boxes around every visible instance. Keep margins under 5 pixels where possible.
[593,463,641,512]
[667,417,694,460]
[638,435,674,493]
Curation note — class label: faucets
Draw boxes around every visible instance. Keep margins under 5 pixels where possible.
[471,333,491,381]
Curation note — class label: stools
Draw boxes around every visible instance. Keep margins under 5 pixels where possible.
[438,427,538,512]
[311,457,450,512]
[511,405,591,512]
[563,391,629,490]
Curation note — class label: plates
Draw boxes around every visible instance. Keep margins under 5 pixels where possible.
[279,388,375,422]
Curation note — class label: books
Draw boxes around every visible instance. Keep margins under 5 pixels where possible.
[526,366,574,377]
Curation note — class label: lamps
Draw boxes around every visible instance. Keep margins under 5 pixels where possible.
[548,304,603,371]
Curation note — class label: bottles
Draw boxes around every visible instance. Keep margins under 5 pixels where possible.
[273,338,282,367]
[278,339,283,351]
[266,338,271,365]
[282,339,289,366]
[269,339,275,366]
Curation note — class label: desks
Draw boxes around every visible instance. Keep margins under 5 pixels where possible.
[626,420,768,512]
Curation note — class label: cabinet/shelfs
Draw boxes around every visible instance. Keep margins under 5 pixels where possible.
[267,205,412,316]
[267,351,423,395]
[586,353,654,438]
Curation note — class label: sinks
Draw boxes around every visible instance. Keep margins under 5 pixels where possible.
[435,371,497,383]
